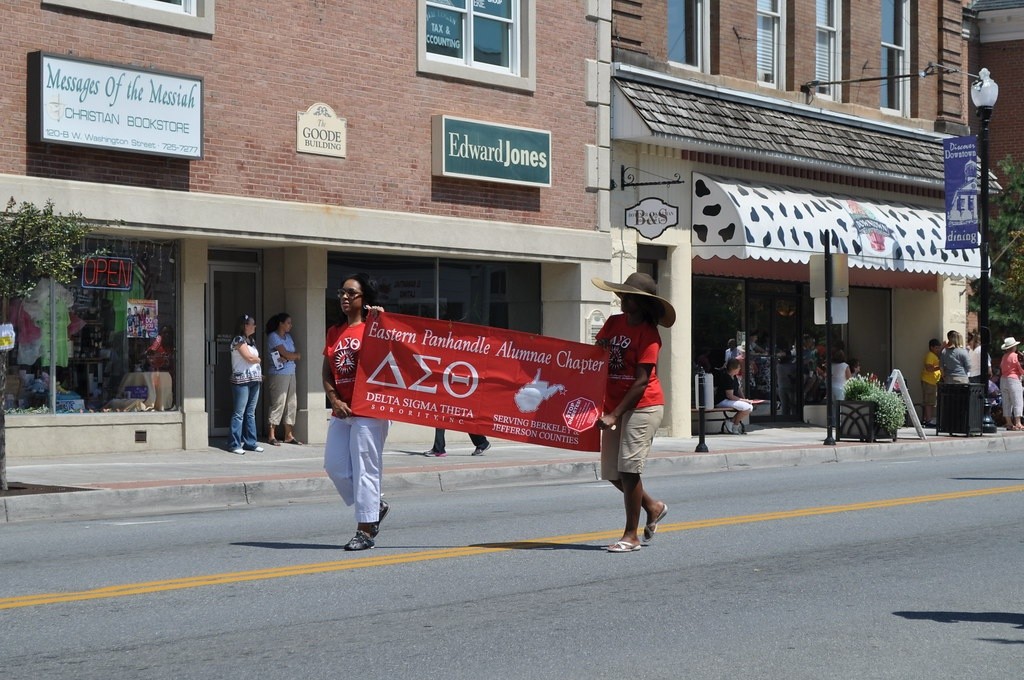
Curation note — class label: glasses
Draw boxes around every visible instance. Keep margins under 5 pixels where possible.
[336,288,363,300]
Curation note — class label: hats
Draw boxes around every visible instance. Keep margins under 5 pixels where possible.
[1001,336,1021,350]
[590,272,677,328]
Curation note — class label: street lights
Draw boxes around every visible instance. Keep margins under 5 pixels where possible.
[970,67,998,431]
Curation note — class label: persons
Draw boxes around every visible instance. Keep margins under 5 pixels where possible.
[128,306,146,337]
[591,272,676,552]
[831,350,860,403]
[1000,337,1024,431]
[714,360,753,435]
[941,329,1006,427]
[265,313,303,446]
[321,274,392,551]
[722,332,830,406]
[922,339,941,428]
[228,315,263,454]
[422,428,492,458]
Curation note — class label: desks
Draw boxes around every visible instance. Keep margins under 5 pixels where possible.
[115,372,173,412]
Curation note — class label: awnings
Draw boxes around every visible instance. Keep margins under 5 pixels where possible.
[692,171,990,278]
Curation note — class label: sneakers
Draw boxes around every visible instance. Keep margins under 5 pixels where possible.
[732,424,741,434]
[423,449,448,458]
[724,419,735,434]
[471,441,492,456]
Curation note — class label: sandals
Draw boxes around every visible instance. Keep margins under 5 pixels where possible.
[1016,424,1024,431]
[1006,424,1020,431]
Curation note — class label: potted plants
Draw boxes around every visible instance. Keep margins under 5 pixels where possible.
[836,372,912,443]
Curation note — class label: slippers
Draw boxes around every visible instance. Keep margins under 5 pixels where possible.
[267,438,281,446]
[606,540,641,553]
[641,503,668,543]
[283,438,303,445]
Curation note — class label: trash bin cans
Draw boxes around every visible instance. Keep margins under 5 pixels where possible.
[936,381,985,437]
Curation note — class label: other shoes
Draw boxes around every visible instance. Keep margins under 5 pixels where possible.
[921,420,937,429]
[231,448,245,455]
[344,529,375,552]
[254,446,264,452]
[370,499,391,539]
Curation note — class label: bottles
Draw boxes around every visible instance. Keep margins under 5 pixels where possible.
[5,393,14,408]
[18,399,24,408]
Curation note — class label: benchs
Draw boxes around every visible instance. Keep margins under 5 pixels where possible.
[691,405,757,434]
